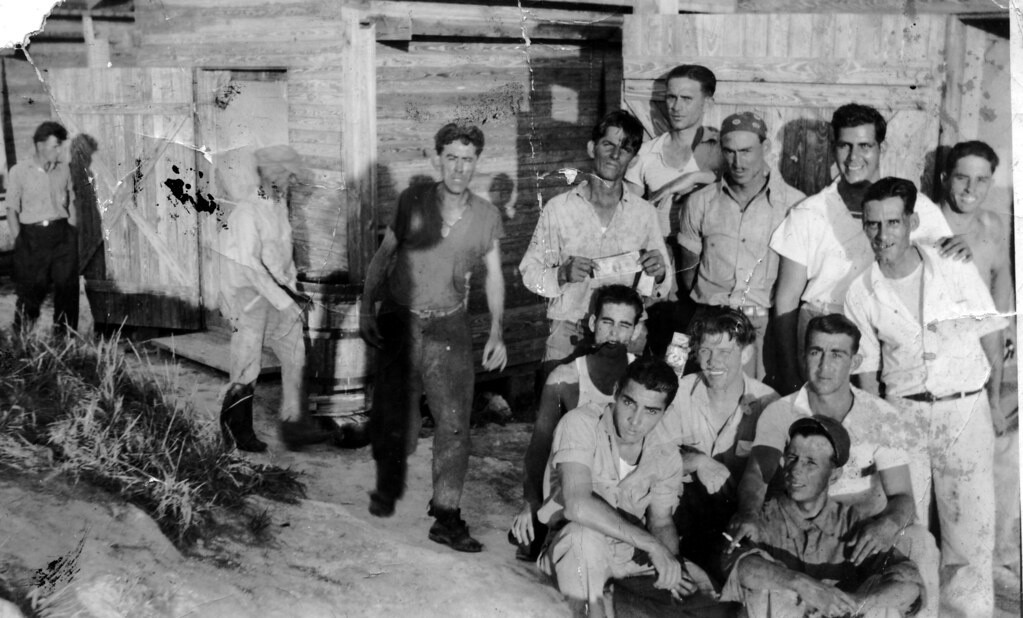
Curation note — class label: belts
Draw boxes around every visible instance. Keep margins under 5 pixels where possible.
[903,389,982,402]
[408,303,463,319]
[36,219,67,227]
[743,307,768,316]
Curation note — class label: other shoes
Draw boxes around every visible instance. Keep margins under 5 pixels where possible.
[428,518,481,552]
[508,511,547,561]
[368,477,404,517]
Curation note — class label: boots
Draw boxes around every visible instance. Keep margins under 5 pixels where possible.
[221,383,267,452]
[280,415,335,452]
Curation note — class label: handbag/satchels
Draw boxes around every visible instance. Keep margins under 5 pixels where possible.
[603,574,747,618]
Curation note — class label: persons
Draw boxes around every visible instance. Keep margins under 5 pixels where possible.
[6,121,82,341]
[517,66,1016,618]
[360,124,507,553]
[222,145,331,450]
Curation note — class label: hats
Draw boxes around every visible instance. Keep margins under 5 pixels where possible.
[788,414,850,466]
[720,112,767,139]
[254,145,302,175]
